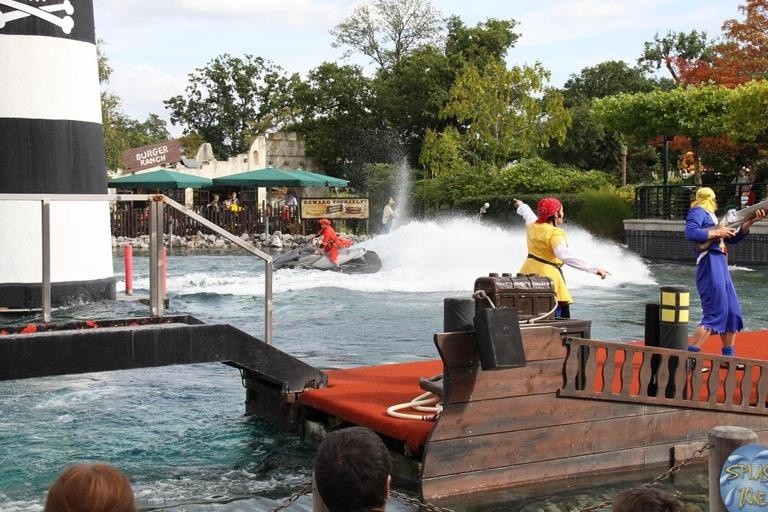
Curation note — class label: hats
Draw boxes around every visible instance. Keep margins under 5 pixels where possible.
[319,218,332,225]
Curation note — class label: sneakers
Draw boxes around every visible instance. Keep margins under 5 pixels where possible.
[690,362,709,373]
[720,361,745,370]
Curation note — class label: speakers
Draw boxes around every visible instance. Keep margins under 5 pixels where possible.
[443,297,476,332]
[472,307,526,371]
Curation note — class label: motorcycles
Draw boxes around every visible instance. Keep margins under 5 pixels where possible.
[272,234,380,273]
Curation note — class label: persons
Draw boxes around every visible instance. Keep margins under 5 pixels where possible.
[308,218,354,273]
[380,197,399,232]
[683,186,766,374]
[513,197,613,318]
[313,425,392,512]
[612,485,685,511]
[42,463,137,512]
[210,190,242,227]
[285,190,299,221]
